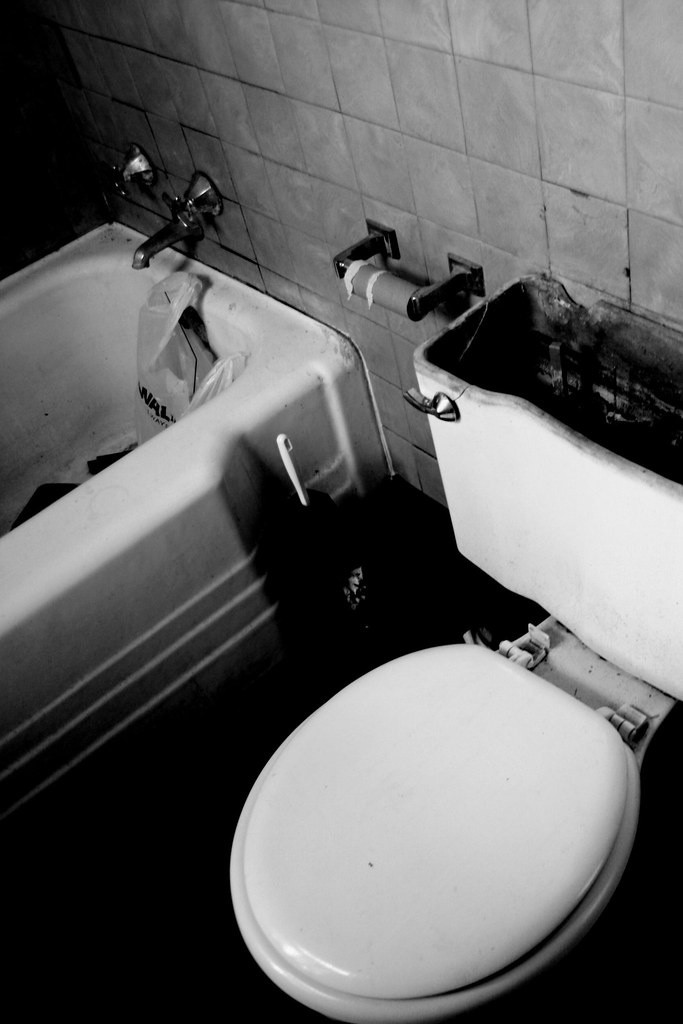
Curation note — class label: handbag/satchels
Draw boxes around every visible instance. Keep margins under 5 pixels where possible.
[134,273,248,446]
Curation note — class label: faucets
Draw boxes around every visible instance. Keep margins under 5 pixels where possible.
[131,214,205,270]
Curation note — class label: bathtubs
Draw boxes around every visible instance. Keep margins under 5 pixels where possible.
[0,222,395,820]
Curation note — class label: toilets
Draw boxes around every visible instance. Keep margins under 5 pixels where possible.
[229,275,683,1024]
